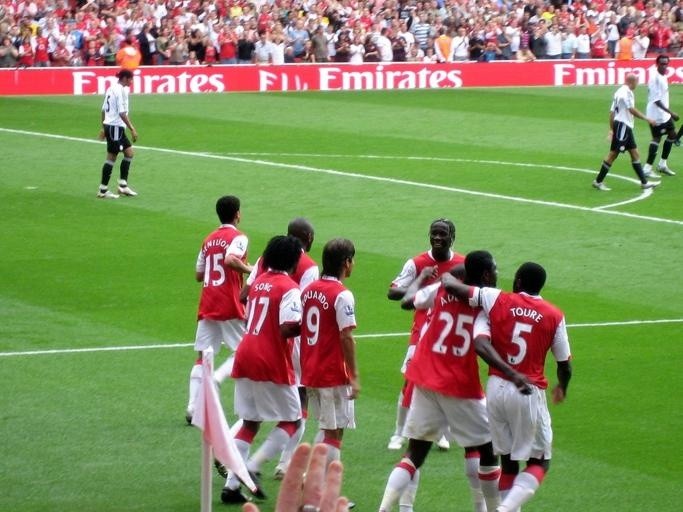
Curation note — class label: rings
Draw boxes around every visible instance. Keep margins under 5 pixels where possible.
[302,504,321,512]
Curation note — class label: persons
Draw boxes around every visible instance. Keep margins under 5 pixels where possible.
[374,249,532,512]
[238,444,359,512]
[1,0,683,63]
[95,69,141,201]
[214,218,324,481]
[221,235,311,507]
[185,194,254,429]
[440,264,573,512]
[637,55,678,179]
[295,239,360,510]
[396,263,494,512]
[386,217,468,452]
[591,73,663,191]
[675,125,683,147]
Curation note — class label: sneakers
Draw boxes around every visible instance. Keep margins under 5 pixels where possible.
[118,186,138,196]
[656,164,676,176]
[96,191,120,199]
[641,180,661,189]
[641,169,662,179]
[183,414,356,508]
[436,440,450,449]
[592,180,613,190]
[387,435,405,449]
